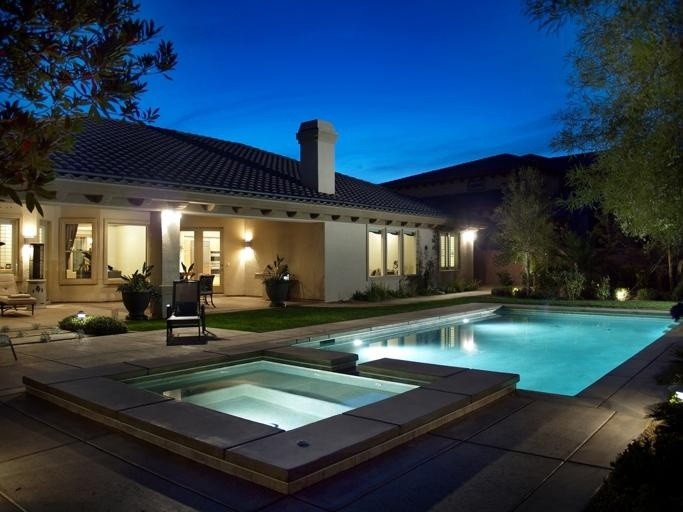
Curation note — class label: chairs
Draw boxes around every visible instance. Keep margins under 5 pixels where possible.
[0,272,35,316]
[165,274,215,343]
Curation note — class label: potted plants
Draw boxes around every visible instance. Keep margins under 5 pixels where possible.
[115,260,158,323]
[261,253,297,309]
